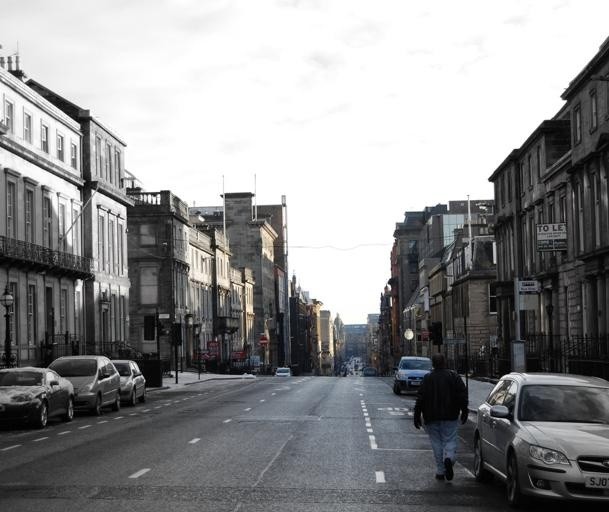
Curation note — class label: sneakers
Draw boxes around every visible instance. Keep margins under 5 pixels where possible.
[436,473,444,480]
[444,458,453,480]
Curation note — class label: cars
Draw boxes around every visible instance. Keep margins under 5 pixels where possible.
[393,356,434,394]
[473,372,609,505]
[109,359,147,408]
[274,367,290,377]
[0,367,76,430]
[47,355,121,416]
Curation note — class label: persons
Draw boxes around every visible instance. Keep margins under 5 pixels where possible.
[415,352,469,480]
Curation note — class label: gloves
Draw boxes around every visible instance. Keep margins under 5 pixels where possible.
[414,411,422,429]
[461,410,469,424]
